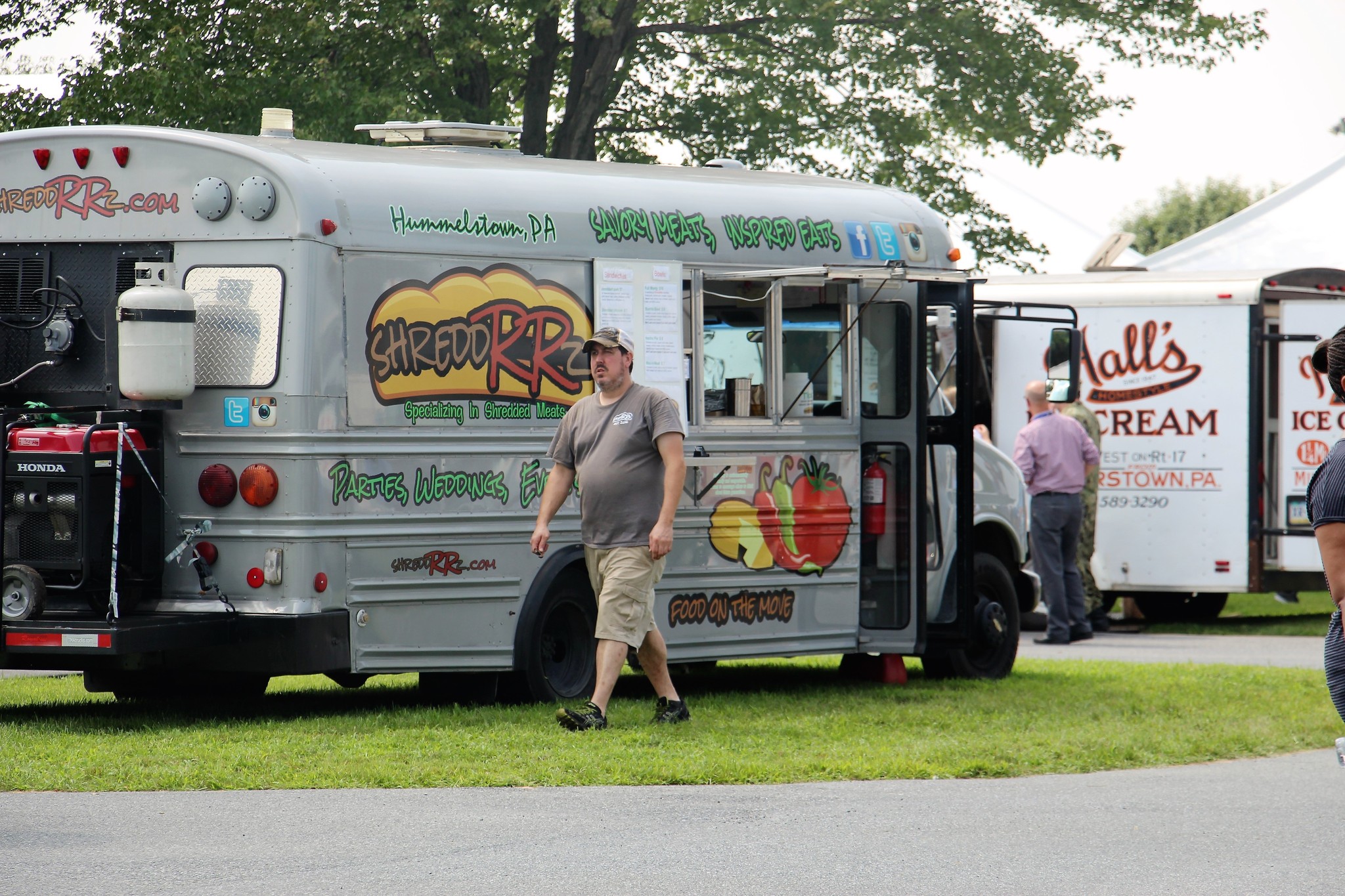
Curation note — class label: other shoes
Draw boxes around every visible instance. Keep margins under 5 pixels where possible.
[1071,630,1092,641]
[1092,619,1110,631]
[1034,638,1070,645]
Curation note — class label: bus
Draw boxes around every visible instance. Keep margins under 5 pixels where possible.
[0,107,1086,719]
[828,267,1345,627]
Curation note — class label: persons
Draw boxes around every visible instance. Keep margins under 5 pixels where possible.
[1306,325,1345,724]
[939,379,1106,644]
[529,326,691,731]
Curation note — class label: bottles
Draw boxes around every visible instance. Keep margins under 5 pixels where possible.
[783,372,814,417]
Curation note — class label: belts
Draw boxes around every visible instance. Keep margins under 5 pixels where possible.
[1035,491,1067,496]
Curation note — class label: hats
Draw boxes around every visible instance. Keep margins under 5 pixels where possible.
[582,327,635,356]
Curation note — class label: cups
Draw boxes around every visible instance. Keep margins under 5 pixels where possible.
[725,378,752,417]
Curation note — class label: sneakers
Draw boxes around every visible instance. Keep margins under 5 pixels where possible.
[555,701,607,732]
[648,696,691,725]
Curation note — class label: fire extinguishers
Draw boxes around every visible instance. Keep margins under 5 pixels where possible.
[861,451,892,535]
